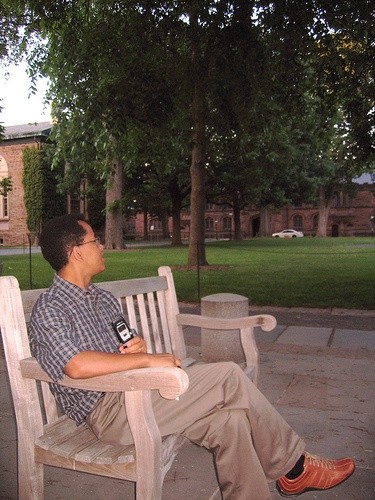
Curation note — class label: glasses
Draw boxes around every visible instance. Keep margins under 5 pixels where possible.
[72,236,101,246]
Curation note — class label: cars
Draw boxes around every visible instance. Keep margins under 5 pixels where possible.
[271,229,304,238]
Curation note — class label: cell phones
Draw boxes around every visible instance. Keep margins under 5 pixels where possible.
[113,316,134,348]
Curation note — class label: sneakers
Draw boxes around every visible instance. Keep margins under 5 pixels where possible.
[276,451,355,498]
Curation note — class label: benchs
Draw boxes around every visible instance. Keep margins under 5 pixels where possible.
[0,266,278,500]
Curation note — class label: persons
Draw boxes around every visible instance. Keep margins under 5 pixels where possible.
[28,212,355,500]
